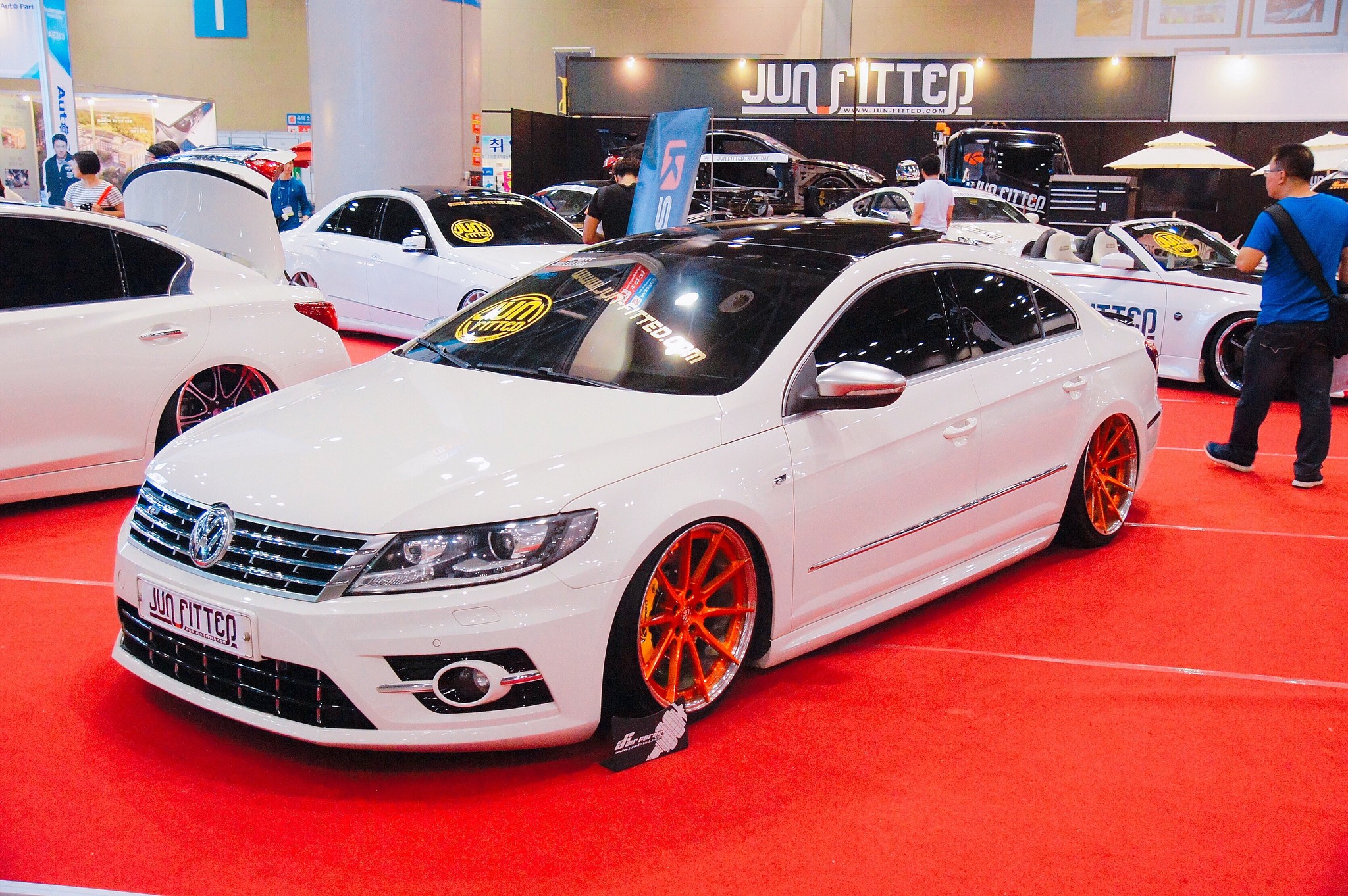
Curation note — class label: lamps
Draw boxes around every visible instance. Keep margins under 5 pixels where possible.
[738,58,748,70]
[858,58,867,67]
[976,57,983,69]
[1111,56,1118,65]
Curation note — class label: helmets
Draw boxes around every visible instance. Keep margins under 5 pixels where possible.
[896,161,919,182]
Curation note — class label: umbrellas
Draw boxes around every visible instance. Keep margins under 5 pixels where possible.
[1103,131,1254,218]
[1249,131,1348,176]
[1310,171,1348,202]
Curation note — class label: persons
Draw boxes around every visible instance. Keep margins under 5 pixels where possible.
[270,159,310,233]
[146,144,174,163]
[5,174,28,187]
[910,155,955,233]
[1203,141,1348,488]
[896,160,920,188]
[63,151,125,218]
[161,140,180,154]
[0,180,23,202]
[582,158,640,245]
[623,145,644,161]
[45,133,81,206]
[486,180,493,188]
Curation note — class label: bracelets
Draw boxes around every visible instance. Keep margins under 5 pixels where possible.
[303,220,307,222]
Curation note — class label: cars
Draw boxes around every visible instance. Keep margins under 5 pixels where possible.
[529,179,734,238]
[607,128,889,220]
[108,221,1161,749]
[277,182,593,341]
[1,203,355,504]
[821,184,1079,259]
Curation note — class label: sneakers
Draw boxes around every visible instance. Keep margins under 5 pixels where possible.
[1291,473,1325,488]
[1204,441,1253,472]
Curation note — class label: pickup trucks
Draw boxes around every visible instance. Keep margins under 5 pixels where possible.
[936,128,1143,228]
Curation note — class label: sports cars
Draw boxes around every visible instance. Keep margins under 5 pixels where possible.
[1019,216,1348,404]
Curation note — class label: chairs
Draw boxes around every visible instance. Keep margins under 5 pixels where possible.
[1045,232,1086,262]
[1091,230,1120,264]
[1136,231,1245,262]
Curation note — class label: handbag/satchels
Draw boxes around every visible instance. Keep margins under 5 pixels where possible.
[1326,297,1348,358]
